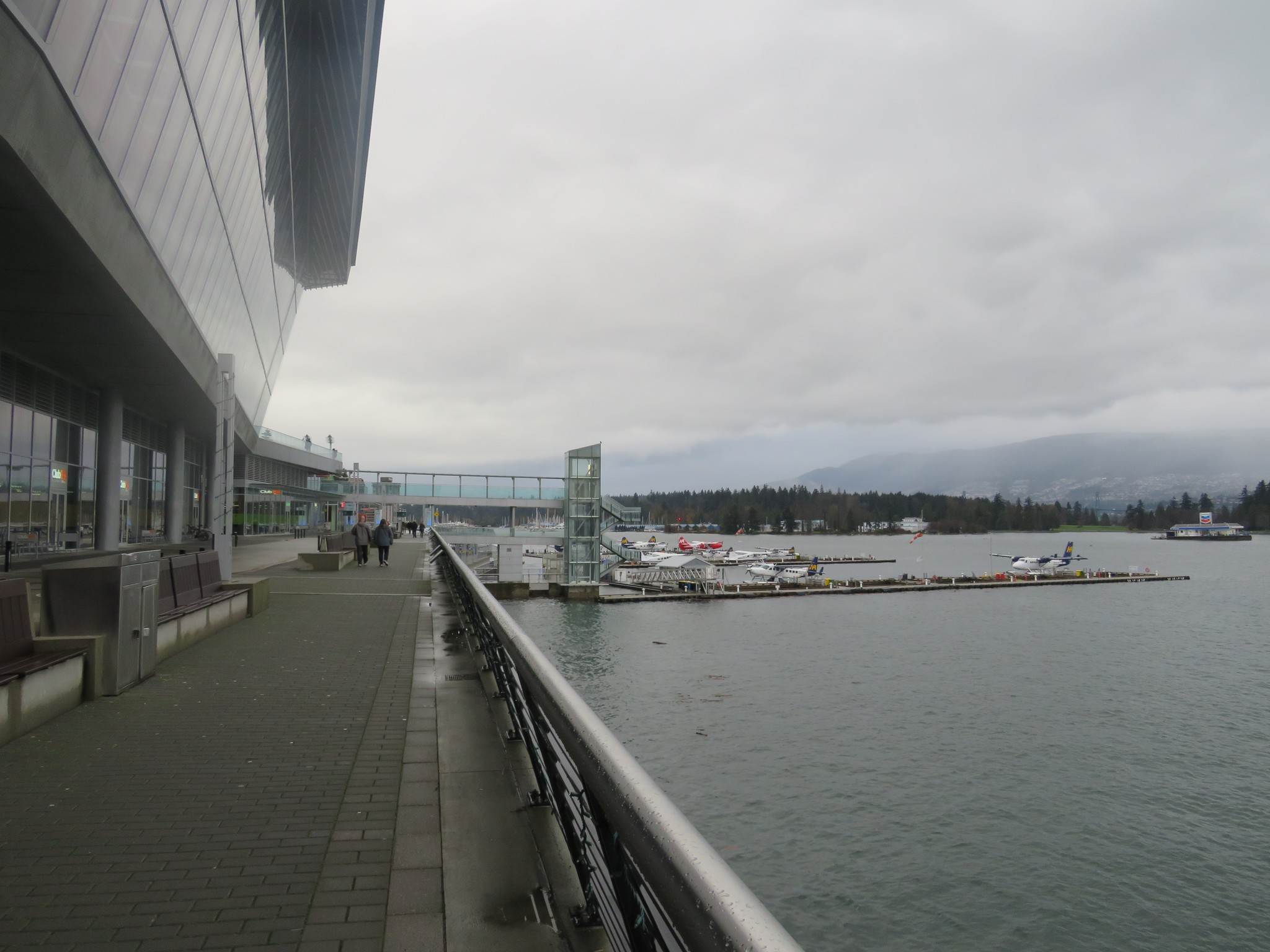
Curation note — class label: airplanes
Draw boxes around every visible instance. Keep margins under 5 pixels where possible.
[679,537,723,550]
[743,557,826,584]
[614,536,668,552]
[639,548,689,561]
[699,546,800,561]
[989,542,1089,575]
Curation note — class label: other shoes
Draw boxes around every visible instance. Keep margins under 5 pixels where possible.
[380,564,383,566]
[384,560,388,566]
[364,559,368,564]
[358,563,362,566]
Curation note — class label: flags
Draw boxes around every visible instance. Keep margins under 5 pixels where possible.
[916,555,923,561]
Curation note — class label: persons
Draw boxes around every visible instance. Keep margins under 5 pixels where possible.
[407,520,412,534]
[374,519,393,566]
[305,438,312,452]
[420,521,426,538]
[332,448,337,459]
[412,521,418,538]
[351,516,372,566]
[387,520,389,526]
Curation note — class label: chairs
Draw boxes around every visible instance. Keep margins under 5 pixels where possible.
[319,533,355,567]
[391,527,402,539]
[0,576,91,747]
[155,550,251,664]
